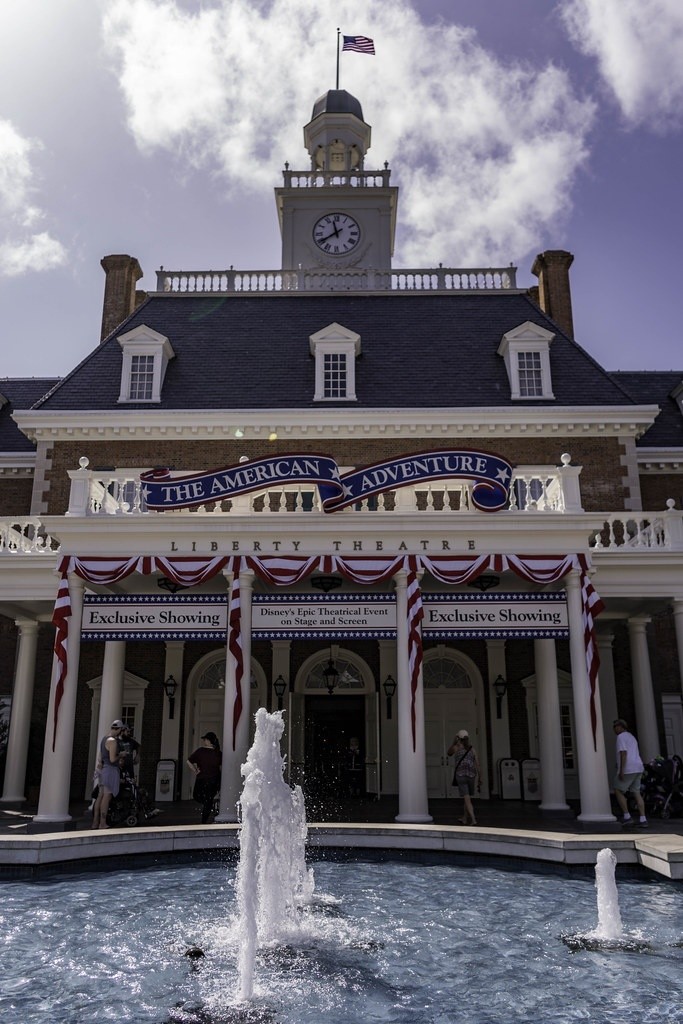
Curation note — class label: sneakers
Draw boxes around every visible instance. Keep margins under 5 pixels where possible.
[634,821,648,827]
[621,817,633,825]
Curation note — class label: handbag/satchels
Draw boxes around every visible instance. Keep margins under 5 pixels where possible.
[452,778,458,786]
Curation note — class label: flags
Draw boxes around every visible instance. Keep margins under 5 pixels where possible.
[341,35,375,55]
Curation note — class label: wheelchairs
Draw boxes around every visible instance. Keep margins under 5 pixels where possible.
[92,780,160,827]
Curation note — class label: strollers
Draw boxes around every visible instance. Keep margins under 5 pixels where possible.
[642,755,683,820]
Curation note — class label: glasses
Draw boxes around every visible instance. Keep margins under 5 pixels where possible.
[477,784,481,793]
[613,723,621,727]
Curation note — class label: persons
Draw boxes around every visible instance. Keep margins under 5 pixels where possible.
[448,729,483,826]
[91,720,162,829]
[186,732,222,824]
[612,719,648,828]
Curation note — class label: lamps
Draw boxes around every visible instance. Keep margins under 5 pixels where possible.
[382,674,397,719]
[322,640,340,696]
[311,576,343,594]
[492,674,507,718]
[273,674,287,710]
[467,575,500,592]
[162,675,178,719]
[159,578,188,593]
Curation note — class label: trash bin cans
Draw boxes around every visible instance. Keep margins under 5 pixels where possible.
[497,758,523,801]
[154,759,180,802]
[519,758,542,801]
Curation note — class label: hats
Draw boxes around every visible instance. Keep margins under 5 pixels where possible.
[121,725,133,731]
[112,720,127,727]
[456,729,468,738]
[201,732,216,739]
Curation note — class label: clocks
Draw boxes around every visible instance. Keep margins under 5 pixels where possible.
[312,212,361,255]
